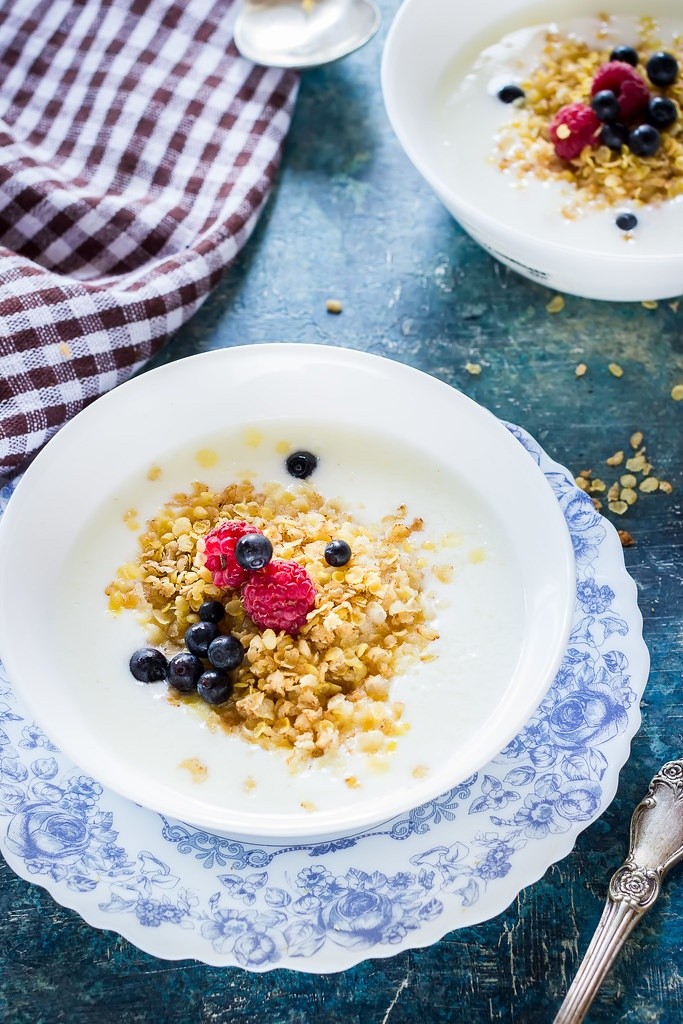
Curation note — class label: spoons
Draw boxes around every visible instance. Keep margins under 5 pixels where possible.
[235,1,380,68]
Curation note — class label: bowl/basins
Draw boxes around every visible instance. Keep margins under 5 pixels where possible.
[380,0,682,303]
[0,342,575,839]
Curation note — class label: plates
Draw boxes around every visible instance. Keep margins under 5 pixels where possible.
[0,423,656,972]
